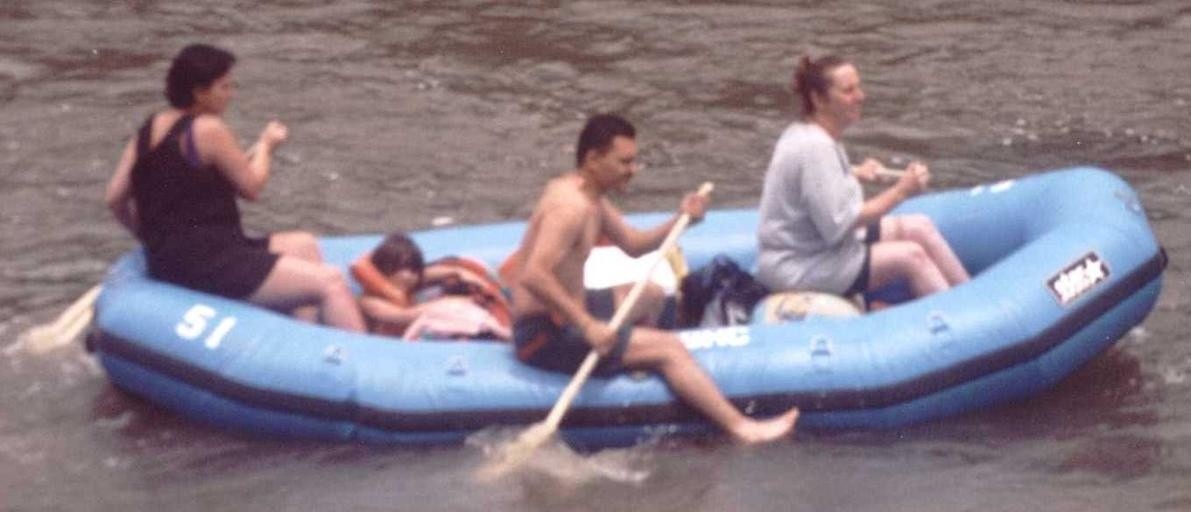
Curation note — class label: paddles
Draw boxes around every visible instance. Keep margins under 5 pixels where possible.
[473,181,713,476]
[31,144,255,351]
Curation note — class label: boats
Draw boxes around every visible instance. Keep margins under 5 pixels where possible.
[91,161,1163,436]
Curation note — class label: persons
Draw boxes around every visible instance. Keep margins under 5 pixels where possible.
[512,113,800,450]
[755,55,971,299]
[357,235,485,338]
[106,44,369,333]
